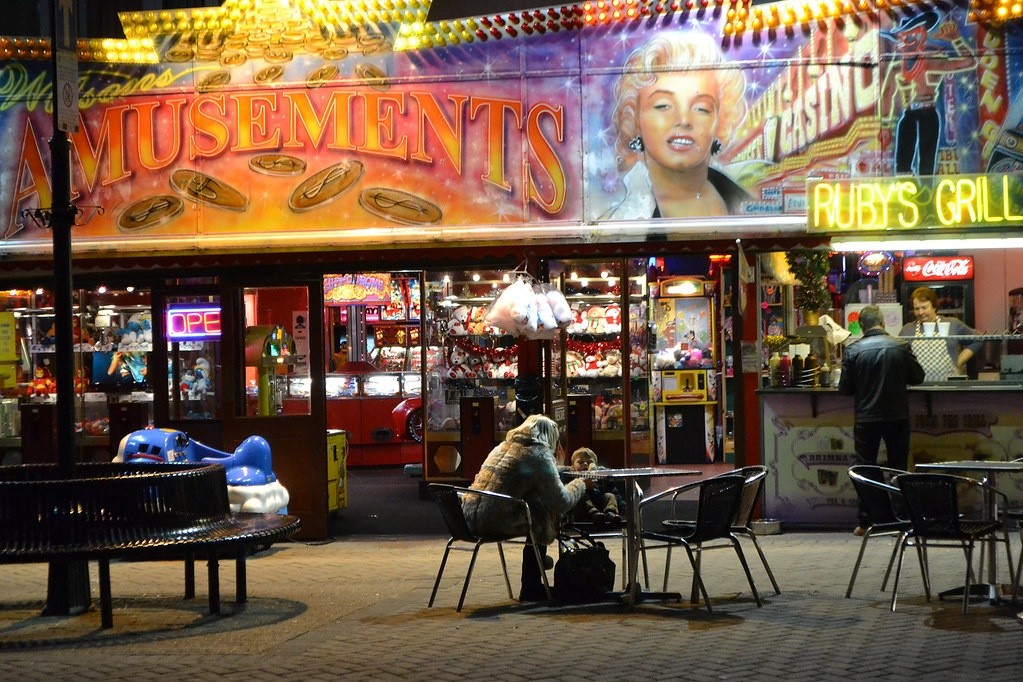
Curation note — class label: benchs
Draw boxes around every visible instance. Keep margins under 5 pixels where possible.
[0,462,303,629]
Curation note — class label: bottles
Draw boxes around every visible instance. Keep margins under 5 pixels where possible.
[806,354,816,384]
[780,352,792,386]
[793,355,803,385]
[770,352,782,386]
[821,363,829,387]
[740,150,880,215]
[831,364,841,386]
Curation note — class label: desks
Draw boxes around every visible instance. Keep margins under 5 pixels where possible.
[916,460,1023,606]
[562,468,703,609]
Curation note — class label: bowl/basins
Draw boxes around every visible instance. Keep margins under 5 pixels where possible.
[751,519,785,534]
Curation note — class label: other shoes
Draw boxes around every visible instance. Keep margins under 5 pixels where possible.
[541,555,553,569]
[518,583,554,601]
[854,526,867,535]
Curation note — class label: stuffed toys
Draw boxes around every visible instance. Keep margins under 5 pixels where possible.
[28,318,110,435]
[113,307,152,347]
[447,303,622,336]
[179,358,210,400]
[594,397,634,431]
[449,349,644,379]
[653,336,713,369]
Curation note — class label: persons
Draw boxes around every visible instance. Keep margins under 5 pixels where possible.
[839,306,925,533]
[460,414,586,601]
[899,287,983,380]
[570,447,622,522]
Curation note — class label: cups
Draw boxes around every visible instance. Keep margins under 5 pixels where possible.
[937,322,950,336]
[923,322,936,337]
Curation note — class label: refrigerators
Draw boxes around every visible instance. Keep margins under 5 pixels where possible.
[894,256,978,381]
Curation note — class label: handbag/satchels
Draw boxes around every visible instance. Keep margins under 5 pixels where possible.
[554,527,617,595]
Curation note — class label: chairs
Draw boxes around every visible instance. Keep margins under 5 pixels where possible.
[560,463,782,614]
[428,482,553,614]
[845,458,1023,612]
